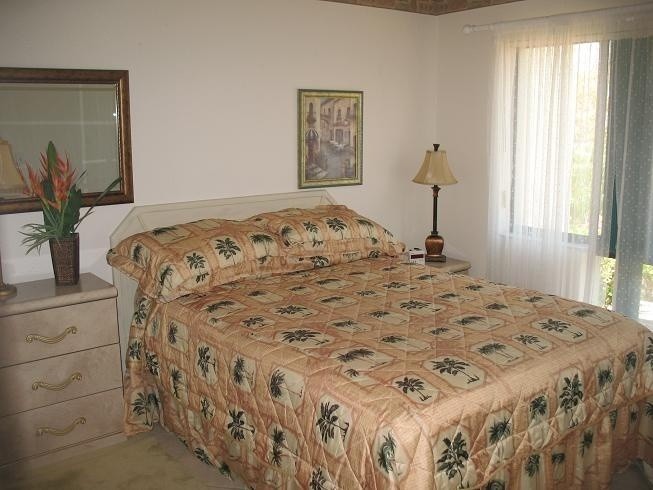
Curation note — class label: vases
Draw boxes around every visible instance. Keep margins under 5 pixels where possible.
[48,232,79,287]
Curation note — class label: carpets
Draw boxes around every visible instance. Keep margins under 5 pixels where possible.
[0,433,206,490]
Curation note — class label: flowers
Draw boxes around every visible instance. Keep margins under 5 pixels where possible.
[13,138,122,255]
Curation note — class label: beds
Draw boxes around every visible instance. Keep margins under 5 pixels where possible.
[108,189,653,490]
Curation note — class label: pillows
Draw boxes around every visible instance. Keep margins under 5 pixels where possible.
[245,204,406,276]
[102,219,316,303]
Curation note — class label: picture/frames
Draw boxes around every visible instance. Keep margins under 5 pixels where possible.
[297,88,363,190]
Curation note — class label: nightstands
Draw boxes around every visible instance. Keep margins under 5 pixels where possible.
[423,254,473,276]
[0,272,128,474]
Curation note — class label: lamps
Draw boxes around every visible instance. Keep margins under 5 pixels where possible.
[412,143,458,263]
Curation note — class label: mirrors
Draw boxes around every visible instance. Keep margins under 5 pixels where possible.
[0,66,133,214]
